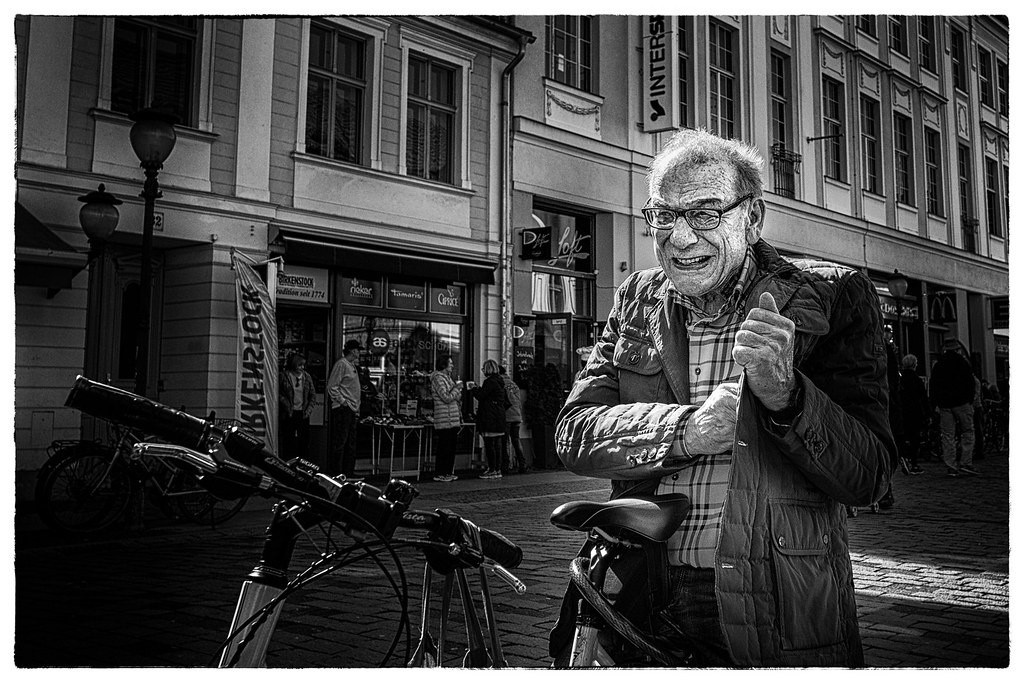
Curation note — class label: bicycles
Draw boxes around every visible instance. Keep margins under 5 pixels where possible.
[63,375,726,668]
[981,400,1008,455]
[920,415,942,458]
[32,420,250,541]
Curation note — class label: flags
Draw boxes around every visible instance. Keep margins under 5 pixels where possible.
[234,256,279,476]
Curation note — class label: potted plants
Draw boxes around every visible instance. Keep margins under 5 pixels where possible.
[524,363,567,468]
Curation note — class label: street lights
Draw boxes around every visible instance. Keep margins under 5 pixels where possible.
[128,104,175,395]
[74,181,125,447]
[886,269,908,369]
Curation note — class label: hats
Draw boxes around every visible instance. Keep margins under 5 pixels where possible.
[942,336,961,350]
[344,339,365,350]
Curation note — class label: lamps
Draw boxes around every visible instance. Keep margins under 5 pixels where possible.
[47,184,124,299]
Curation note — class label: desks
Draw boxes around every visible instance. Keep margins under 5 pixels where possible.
[362,422,477,482]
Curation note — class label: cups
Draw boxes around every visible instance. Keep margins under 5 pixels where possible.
[455,380,463,392]
[466,381,475,390]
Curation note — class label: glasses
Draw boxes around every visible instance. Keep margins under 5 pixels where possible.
[641,193,749,231]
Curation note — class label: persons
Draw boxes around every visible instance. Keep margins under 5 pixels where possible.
[982,379,1002,428]
[430,353,464,482]
[553,128,898,668]
[497,365,528,475]
[325,339,365,481]
[899,354,940,475]
[469,360,506,479]
[932,336,982,476]
[279,354,316,464]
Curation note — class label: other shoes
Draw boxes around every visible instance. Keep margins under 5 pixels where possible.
[950,470,959,475]
[960,466,982,476]
[478,469,502,478]
[433,473,458,482]
[911,465,925,475]
[900,456,909,476]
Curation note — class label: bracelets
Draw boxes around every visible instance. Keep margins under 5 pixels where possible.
[771,386,803,418]
